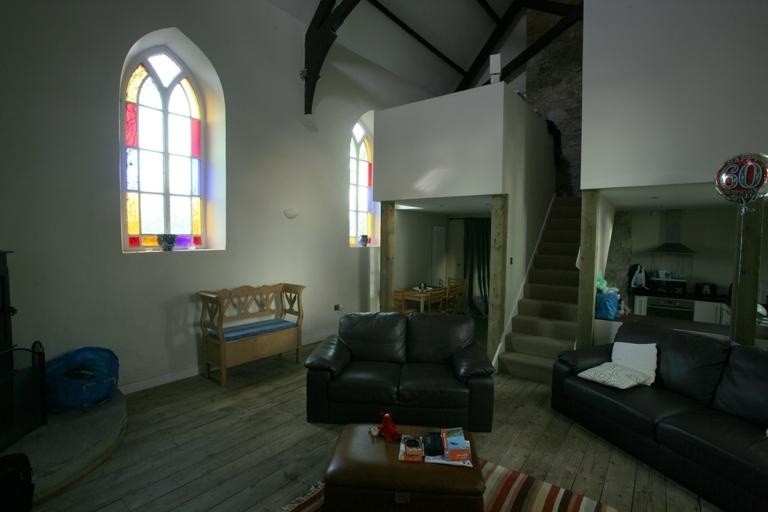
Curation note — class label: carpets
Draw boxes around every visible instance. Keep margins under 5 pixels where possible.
[279,455,619,512]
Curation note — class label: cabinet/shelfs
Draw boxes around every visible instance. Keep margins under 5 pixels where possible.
[634,291,768,340]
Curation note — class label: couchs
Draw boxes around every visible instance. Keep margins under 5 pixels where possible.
[306,312,494,433]
[552,320,768,512]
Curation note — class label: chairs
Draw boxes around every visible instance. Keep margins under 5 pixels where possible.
[393,277,465,316]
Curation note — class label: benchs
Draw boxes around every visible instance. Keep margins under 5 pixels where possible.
[199,284,305,387]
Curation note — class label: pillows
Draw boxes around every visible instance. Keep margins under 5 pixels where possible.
[578,362,648,389]
[611,341,659,386]
